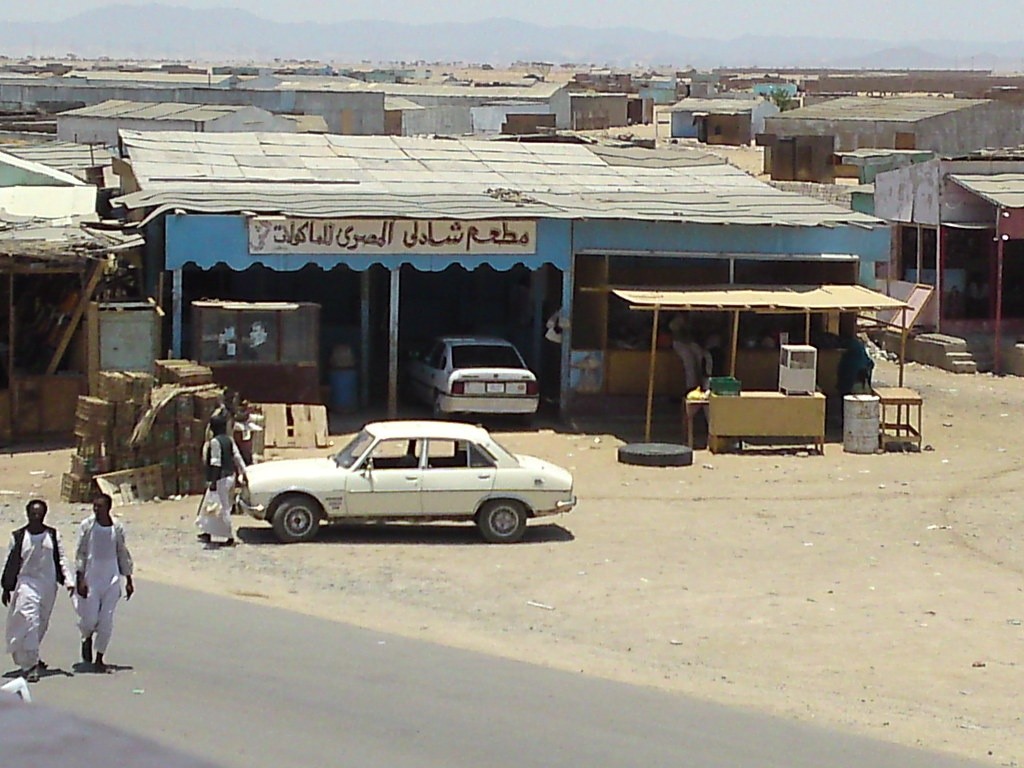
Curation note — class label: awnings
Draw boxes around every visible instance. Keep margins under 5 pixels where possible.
[612,285,912,442]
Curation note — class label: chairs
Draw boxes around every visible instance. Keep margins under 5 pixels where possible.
[455,450,468,467]
[396,453,418,468]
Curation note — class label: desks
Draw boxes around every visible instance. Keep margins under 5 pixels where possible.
[707,391,827,455]
[680,395,743,451]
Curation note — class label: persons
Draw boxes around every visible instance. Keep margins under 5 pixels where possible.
[195,388,265,546]
[71,494,135,675]
[0,499,76,683]
[837,350,877,435]
[327,342,359,415]
[657,337,717,449]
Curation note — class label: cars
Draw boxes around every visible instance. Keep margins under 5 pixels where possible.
[406,335,540,424]
[234,422,576,545]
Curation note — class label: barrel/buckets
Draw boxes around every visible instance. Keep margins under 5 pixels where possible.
[843,393,880,454]
[329,361,358,410]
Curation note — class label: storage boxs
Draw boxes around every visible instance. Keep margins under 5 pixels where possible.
[708,376,742,396]
[61,359,267,502]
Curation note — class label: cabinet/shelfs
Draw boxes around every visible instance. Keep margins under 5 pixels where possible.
[778,344,817,397]
[872,387,923,453]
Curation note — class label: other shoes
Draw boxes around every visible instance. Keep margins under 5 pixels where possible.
[82,638,93,662]
[195,533,210,542]
[225,538,234,545]
[27,666,39,682]
[37,659,48,670]
[95,663,116,674]
